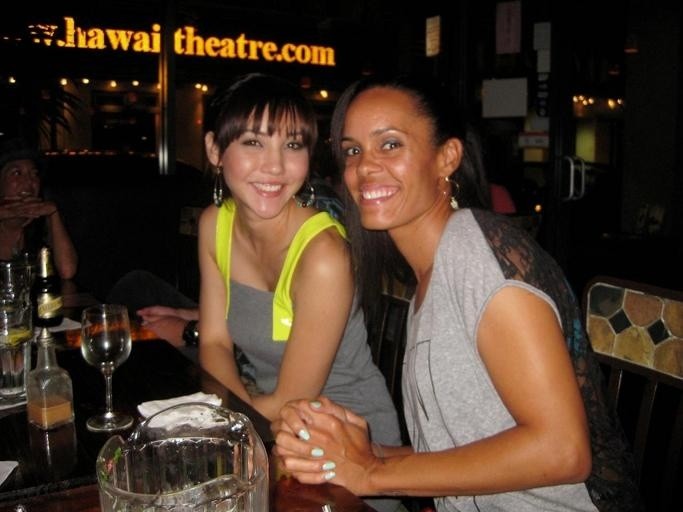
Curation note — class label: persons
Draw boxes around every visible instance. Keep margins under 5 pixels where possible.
[197,70,403,509]
[272,67,636,512]
[1,147,79,281]
[135,306,203,351]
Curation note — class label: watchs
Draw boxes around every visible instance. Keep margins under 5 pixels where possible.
[183,318,198,347]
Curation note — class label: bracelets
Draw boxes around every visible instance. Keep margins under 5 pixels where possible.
[48,208,59,217]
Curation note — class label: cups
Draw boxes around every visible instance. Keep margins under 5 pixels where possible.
[95,402,270,512]
[0,252,34,410]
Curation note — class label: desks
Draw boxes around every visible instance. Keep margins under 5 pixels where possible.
[0,268,378,511]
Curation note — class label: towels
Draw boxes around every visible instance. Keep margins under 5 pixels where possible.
[136,393,227,431]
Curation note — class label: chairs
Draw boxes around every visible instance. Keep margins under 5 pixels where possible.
[583,275,683,472]
[368,274,415,395]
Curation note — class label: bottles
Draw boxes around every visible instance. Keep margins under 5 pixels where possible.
[28,421,77,465]
[29,245,65,329]
[25,326,76,430]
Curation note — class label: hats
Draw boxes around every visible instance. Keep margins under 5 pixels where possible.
[0,136,41,168]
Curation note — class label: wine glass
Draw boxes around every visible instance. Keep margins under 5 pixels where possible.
[79,303,134,434]
